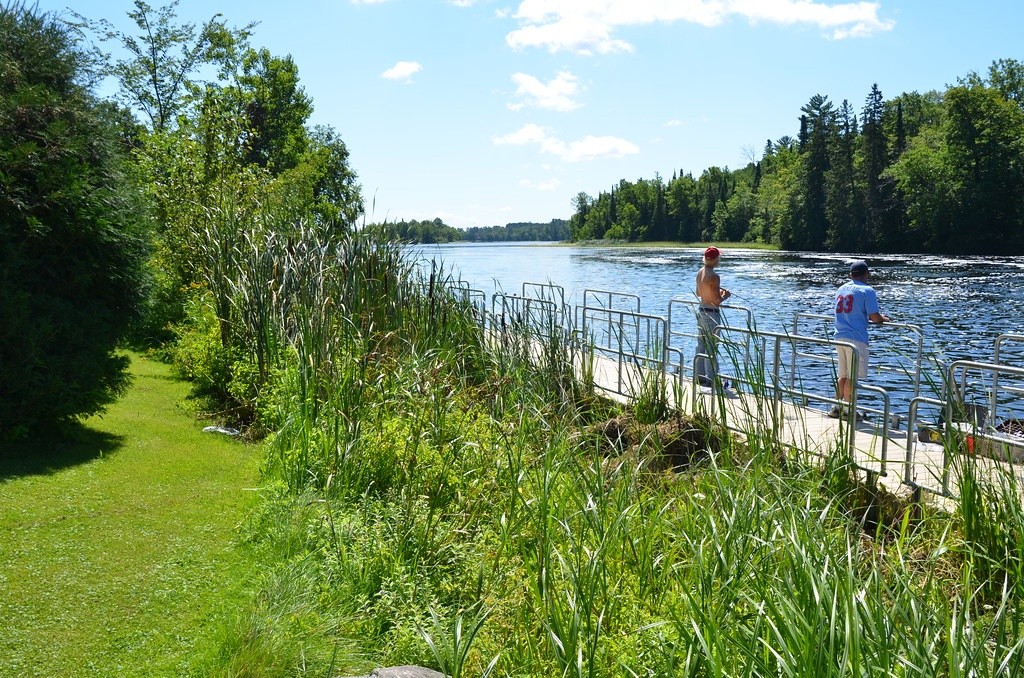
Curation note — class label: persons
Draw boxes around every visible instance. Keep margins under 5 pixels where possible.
[691,243,730,387]
[825,260,892,424]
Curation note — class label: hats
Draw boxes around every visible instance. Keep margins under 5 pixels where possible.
[849,261,875,274]
[704,246,720,260]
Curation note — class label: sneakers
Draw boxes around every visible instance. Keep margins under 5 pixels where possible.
[706,381,723,387]
[696,378,707,384]
[828,405,849,418]
[840,409,866,421]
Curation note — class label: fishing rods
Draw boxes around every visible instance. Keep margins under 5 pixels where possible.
[720,286,768,310]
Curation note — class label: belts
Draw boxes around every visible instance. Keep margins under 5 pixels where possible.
[698,307,720,312]
